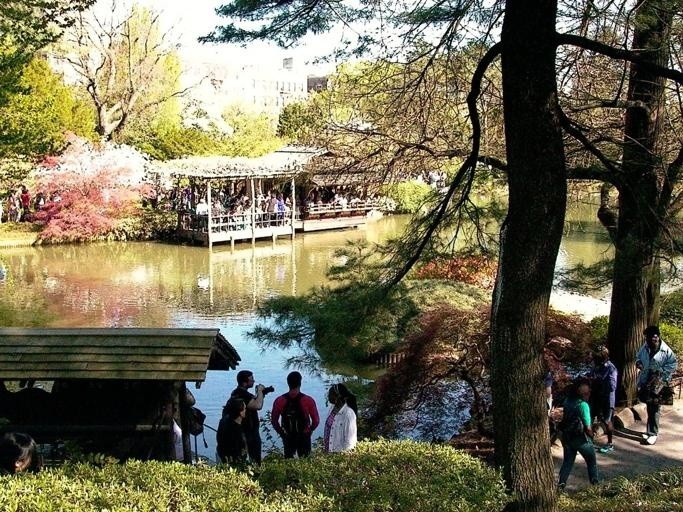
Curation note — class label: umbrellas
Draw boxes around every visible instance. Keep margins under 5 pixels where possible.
[184,404,208,449]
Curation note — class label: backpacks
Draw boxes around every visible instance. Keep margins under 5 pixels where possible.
[280,392,307,437]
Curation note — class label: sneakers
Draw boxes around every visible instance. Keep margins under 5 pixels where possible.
[599,443,614,453]
[640,433,649,440]
[647,436,658,444]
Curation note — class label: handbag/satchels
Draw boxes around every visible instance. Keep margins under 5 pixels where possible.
[637,369,675,405]
[557,398,585,441]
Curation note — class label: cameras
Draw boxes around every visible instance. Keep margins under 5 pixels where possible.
[256,385,275,397]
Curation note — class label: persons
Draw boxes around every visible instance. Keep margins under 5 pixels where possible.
[634,325,677,446]
[5,433,41,475]
[323,382,359,453]
[5,186,59,224]
[270,370,320,457]
[231,370,265,466]
[557,381,600,489]
[216,398,248,465]
[168,179,366,233]
[184,388,196,407]
[544,361,558,447]
[582,344,616,453]
